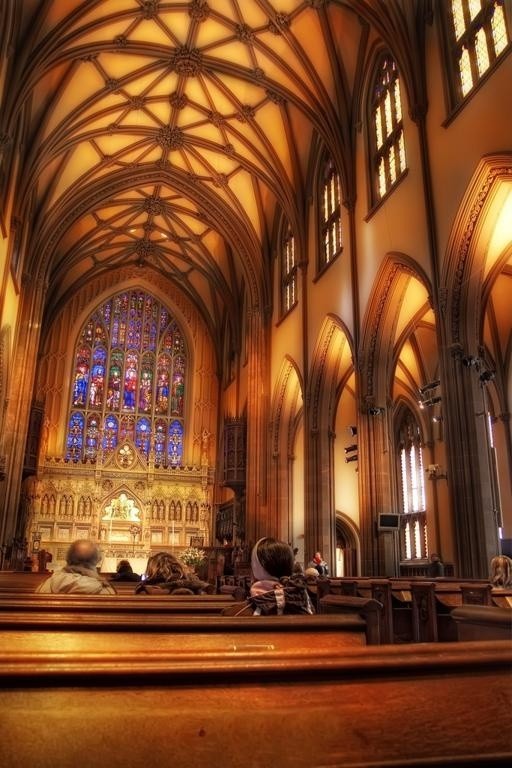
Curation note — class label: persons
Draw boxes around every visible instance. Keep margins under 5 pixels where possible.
[110,561,141,582]
[135,552,216,596]
[427,552,444,577]
[307,551,330,576]
[488,555,512,590]
[35,539,117,594]
[220,537,316,616]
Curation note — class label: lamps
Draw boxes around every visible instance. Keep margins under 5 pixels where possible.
[339,345,499,483]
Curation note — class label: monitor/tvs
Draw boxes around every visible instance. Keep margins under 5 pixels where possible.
[377,513,400,531]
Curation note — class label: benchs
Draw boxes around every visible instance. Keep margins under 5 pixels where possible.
[1,571,512,767]
